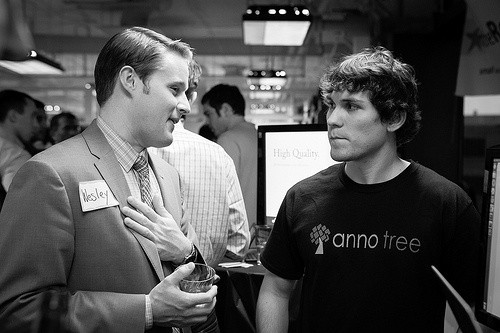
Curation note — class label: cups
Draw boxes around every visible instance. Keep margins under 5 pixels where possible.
[174,263,216,306]
[253,225,272,265]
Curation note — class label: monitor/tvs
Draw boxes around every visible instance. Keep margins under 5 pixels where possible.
[475,144,500,332]
[256,123,345,227]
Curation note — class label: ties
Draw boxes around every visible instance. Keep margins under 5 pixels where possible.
[132,155,156,213]
